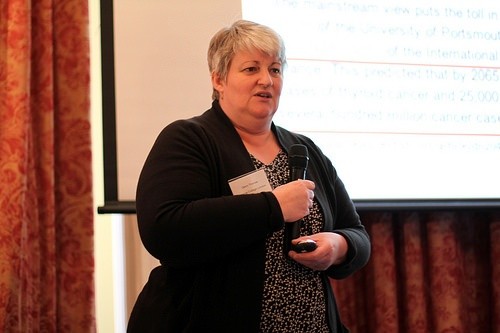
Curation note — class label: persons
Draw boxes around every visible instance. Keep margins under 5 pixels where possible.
[125,16,372,332]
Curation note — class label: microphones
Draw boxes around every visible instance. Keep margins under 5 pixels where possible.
[282,144,310,253]
